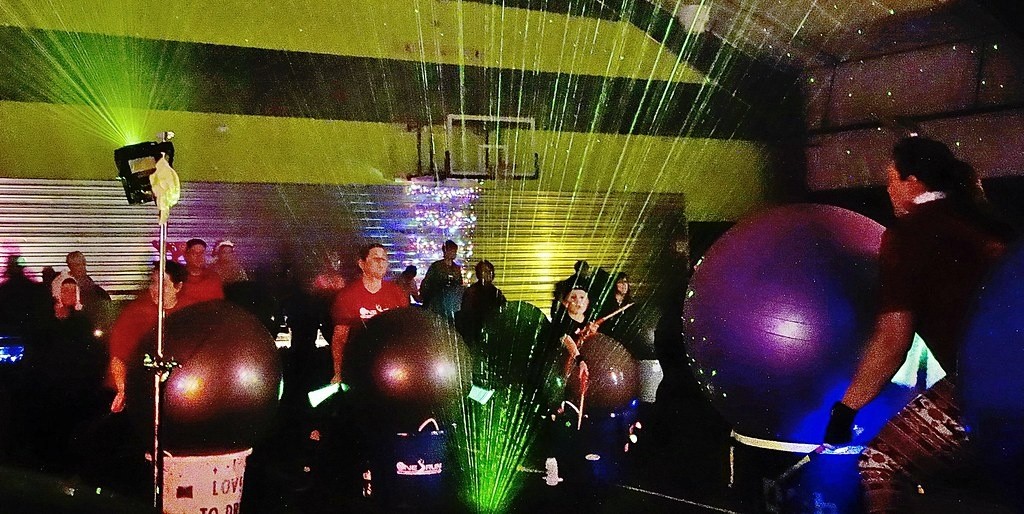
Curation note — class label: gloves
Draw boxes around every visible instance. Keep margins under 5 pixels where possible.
[826,403,851,449]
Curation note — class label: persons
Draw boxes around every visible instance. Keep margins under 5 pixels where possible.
[37,238,638,498]
[822,138,1017,514]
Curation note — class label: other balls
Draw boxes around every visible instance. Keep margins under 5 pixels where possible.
[566,333,638,412]
[343,307,473,428]
[129,298,282,445]
[681,201,946,446]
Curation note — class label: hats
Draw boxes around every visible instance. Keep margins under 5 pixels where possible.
[52,267,81,311]
